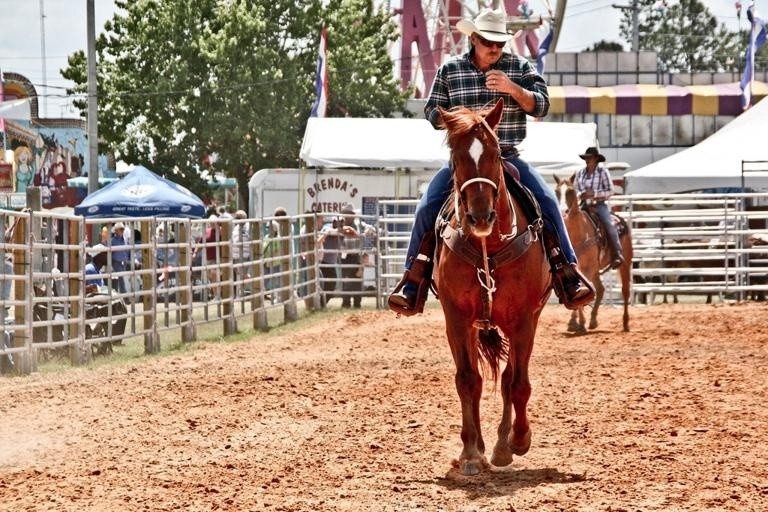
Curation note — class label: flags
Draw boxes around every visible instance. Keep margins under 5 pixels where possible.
[735,1,768,110]
[308,26,331,118]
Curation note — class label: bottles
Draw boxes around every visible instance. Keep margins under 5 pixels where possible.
[341,202,359,213]
[454,7,515,43]
[112,222,125,231]
[579,146,606,162]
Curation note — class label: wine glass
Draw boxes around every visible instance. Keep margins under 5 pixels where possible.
[492,80,496,85]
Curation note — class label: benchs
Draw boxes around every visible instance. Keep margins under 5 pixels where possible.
[735,1,768,110]
[308,26,331,118]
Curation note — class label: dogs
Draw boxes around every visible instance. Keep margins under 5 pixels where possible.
[429,93,552,478]
[662,237,767,303]
[553,171,634,335]
[637,237,669,305]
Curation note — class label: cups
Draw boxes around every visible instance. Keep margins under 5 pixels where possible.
[476,34,508,49]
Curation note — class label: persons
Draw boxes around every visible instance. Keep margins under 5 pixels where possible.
[110,222,132,305]
[133,223,204,290]
[572,147,624,271]
[388,9,592,315]
[230,210,253,302]
[192,214,225,302]
[300,205,370,308]
[85,244,127,347]
[261,208,295,300]
[717,201,740,242]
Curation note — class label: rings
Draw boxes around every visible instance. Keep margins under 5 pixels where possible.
[492,80,496,85]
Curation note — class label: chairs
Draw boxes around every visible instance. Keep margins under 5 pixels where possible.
[567,274,589,301]
[390,288,416,309]
[613,258,621,267]
